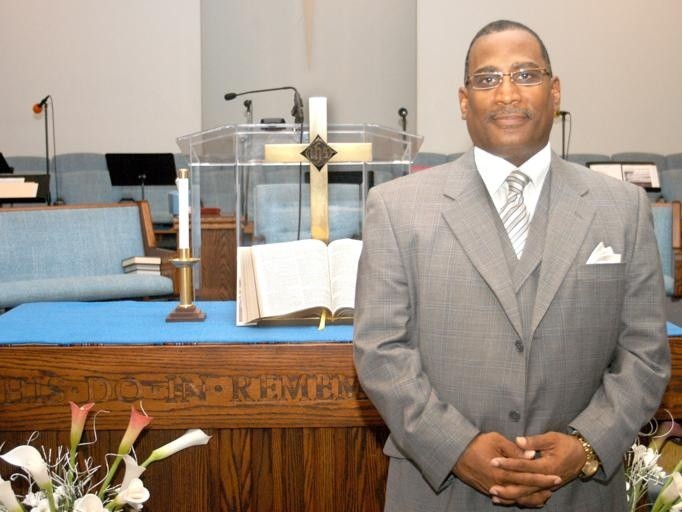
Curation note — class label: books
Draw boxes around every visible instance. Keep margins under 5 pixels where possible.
[234,237,363,327]
[121,254,162,276]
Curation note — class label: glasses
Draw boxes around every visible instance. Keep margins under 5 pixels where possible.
[461,67,554,87]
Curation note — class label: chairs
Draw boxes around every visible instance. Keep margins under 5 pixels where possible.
[559,150,682,301]
[4,152,191,251]
[202,149,466,243]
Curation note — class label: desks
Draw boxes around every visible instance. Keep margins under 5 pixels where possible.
[0,300,682,512]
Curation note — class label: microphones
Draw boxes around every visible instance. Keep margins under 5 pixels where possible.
[33,96,51,113]
[243,99,253,107]
[224,86,304,123]
[398,107,408,118]
[554,109,569,118]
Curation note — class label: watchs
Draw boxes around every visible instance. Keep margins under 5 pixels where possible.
[573,430,599,481]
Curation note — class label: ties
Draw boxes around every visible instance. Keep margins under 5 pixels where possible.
[496,170,532,258]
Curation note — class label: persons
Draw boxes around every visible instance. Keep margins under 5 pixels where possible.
[350,18,672,511]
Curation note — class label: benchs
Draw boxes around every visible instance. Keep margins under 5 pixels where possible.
[0,201,210,316]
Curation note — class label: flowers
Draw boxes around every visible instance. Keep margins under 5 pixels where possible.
[623,408,682,512]
[0,399,214,512]
